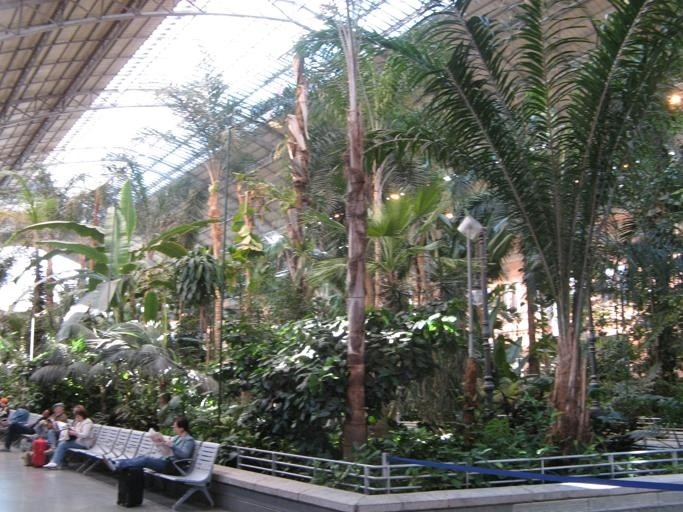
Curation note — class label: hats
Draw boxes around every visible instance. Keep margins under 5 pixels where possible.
[51,402,65,407]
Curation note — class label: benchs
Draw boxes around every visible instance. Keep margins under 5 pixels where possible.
[10,412,222,512]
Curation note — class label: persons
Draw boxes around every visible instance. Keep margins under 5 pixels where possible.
[0,398,97,471]
[102,416,196,475]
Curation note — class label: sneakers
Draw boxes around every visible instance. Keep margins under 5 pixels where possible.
[42,462,61,470]
[103,454,119,471]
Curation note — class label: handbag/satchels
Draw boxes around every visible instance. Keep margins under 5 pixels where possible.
[21,450,35,466]
[165,454,190,474]
[59,430,76,441]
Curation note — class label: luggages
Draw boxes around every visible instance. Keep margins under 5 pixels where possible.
[116,465,145,508]
[32,435,49,468]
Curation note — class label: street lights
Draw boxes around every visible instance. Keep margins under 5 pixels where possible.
[456,213,480,361]
